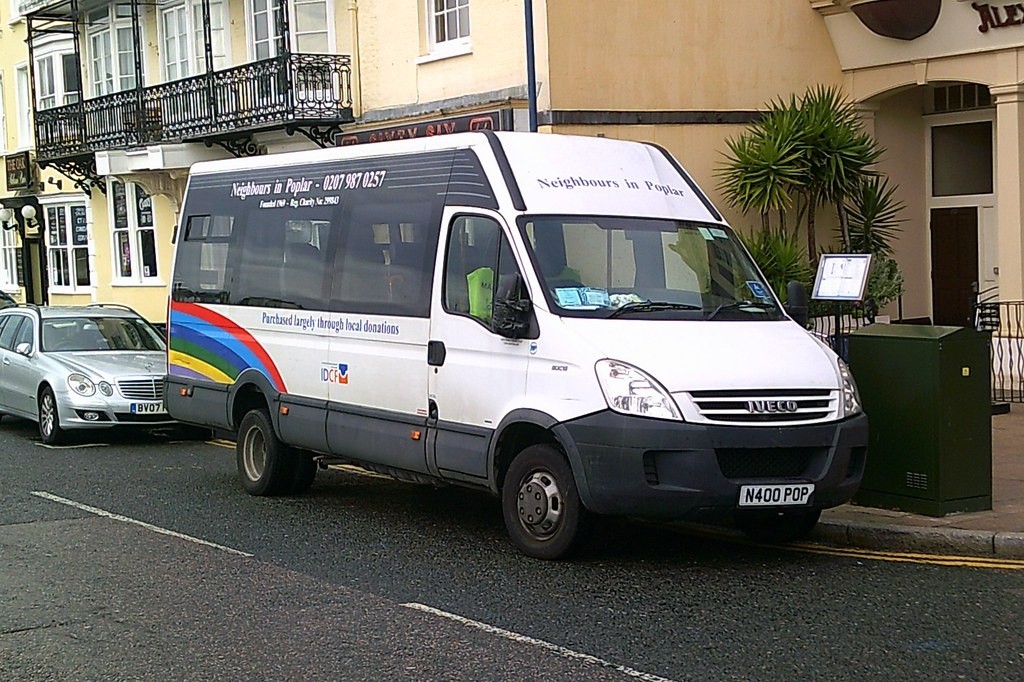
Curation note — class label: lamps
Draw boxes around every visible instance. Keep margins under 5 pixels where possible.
[0,208,12,223]
[21,205,36,222]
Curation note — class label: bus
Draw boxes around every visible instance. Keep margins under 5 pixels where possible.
[161,131,866,562]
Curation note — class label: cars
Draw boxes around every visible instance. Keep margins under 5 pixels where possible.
[0,302,217,446]
